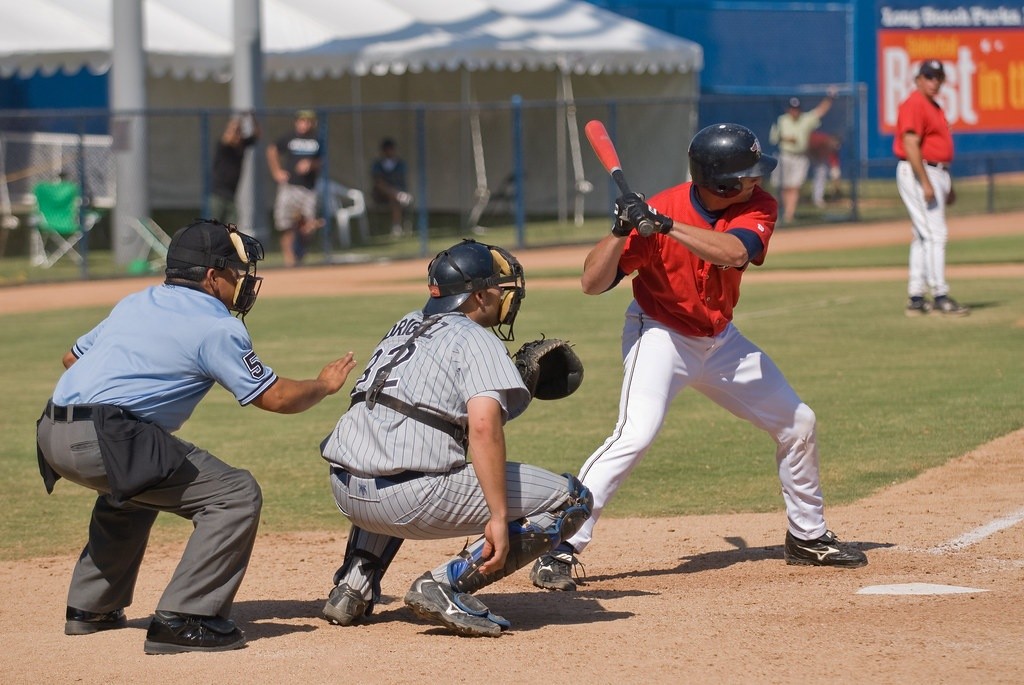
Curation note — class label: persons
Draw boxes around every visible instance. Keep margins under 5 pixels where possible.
[894,58,970,316]
[769,84,835,225]
[265,108,321,268]
[35,221,357,655]
[322,239,595,636]
[530,123,869,592]
[204,111,261,227]
[373,135,414,238]
[805,129,845,209]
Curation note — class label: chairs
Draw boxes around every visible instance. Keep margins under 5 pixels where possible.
[25,177,101,270]
[485,169,531,225]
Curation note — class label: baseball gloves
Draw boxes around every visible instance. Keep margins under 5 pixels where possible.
[947,187,955,204]
[510,331,585,400]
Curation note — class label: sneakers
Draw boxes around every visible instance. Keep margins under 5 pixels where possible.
[405,571,501,637]
[785,530,867,567]
[530,551,586,591]
[322,583,370,625]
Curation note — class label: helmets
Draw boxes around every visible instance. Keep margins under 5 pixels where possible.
[688,122,778,198]
[422,242,501,316]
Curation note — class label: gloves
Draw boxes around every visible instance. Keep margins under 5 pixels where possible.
[611,193,645,236]
[626,197,674,234]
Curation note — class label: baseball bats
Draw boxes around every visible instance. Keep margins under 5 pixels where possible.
[584,119,656,237]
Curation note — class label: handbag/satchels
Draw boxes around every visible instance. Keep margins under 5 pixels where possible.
[93,404,189,502]
[37,414,62,494]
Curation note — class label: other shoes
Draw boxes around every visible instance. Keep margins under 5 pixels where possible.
[932,299,969,317]
[904,300,933,316]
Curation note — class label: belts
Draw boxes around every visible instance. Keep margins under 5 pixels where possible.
[46,401,92,421]
[333,468,424,489]
[928,163,946,170]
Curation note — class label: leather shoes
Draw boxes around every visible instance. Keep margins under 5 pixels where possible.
[65,605,126,635]
[144,612,246,654]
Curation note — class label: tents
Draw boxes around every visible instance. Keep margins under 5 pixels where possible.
[0,0,703,219]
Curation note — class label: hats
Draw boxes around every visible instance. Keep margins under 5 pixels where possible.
[919,59,944,77]
[167,222,250,269]
